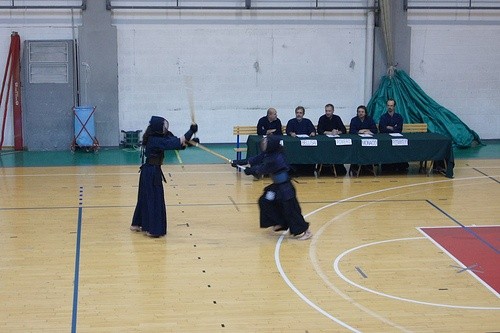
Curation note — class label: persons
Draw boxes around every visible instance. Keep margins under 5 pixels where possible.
[286,107,315,137]
[349,105,378,171]
[231,134,312,241]
[130,115,200,238]
[257,108,283,135]
[317,104,347,174]
[379,99,409,170]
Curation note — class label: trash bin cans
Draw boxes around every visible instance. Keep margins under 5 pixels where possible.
[73,106,96,147]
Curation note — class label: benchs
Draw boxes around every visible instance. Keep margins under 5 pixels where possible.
[231,124,428,174]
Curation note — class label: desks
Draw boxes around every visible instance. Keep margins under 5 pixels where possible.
[244,132,452,178]
